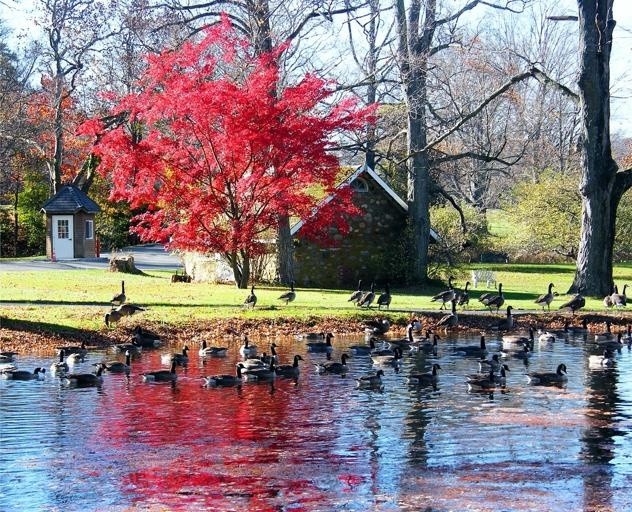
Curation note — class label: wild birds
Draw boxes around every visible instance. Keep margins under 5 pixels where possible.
[1,325,350,392]
[611,285,627,308]
[244,284,257,310]
[459,281,471,307]
[485,282,505,313]
[357,281,377,309]
[348,279,363,307]
[277,280,296,306]
[109,280,126,308]
[376,282,391,309]
[111,304,145,323]
[534,283,555,312]
[351,299,632,393]
[431,276,456,310]
[559,286,586,314]
[104,308,121,327]
[479,292,498,308]
[603,284,619,307]
